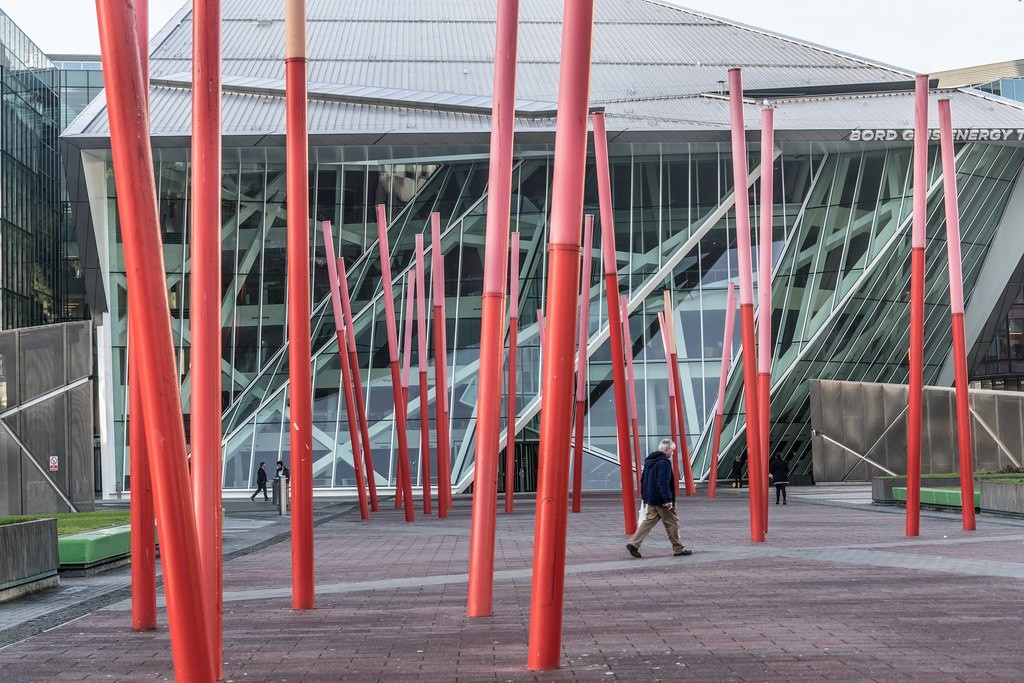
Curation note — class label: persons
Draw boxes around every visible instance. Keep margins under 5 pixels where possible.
[770,453,790,505]
[274,461,290,483]
[250,462,271,501]
[733,456,742,488]
[626,439,693,558]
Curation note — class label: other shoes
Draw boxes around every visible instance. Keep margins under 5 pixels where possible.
[783,500,786,504]
[776,502,779,504]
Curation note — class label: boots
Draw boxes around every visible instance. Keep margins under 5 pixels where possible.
[739,484,742,488]
[734,484,738,488]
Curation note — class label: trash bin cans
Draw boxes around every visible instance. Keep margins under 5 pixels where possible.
[272,476,289,507]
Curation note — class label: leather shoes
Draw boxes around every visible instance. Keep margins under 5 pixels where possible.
[672,549,693,555]
[627,543,641,557]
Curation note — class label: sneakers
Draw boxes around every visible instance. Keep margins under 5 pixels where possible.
[264,498,271,501]
[251,497,255,501]
